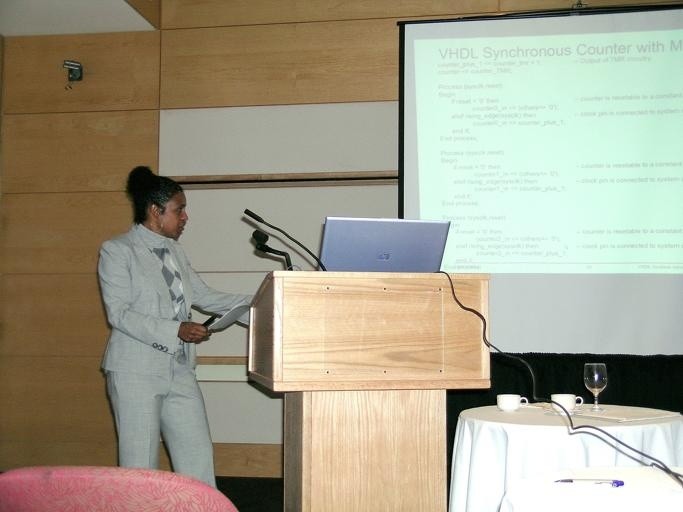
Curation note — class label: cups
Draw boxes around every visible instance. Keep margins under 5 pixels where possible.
[496,394,528,411]
[551,394,584,416]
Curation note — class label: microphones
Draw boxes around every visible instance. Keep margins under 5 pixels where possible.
[243,208,327,271]
[251,230,293,271]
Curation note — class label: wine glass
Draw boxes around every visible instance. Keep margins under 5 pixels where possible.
[583,364,607,414]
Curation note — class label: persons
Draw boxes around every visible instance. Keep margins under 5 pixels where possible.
[98,165,254,492]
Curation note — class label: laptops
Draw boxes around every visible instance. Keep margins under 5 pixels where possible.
[318,218,450,272]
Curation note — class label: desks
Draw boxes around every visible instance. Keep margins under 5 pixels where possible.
[458,404,682,512]
